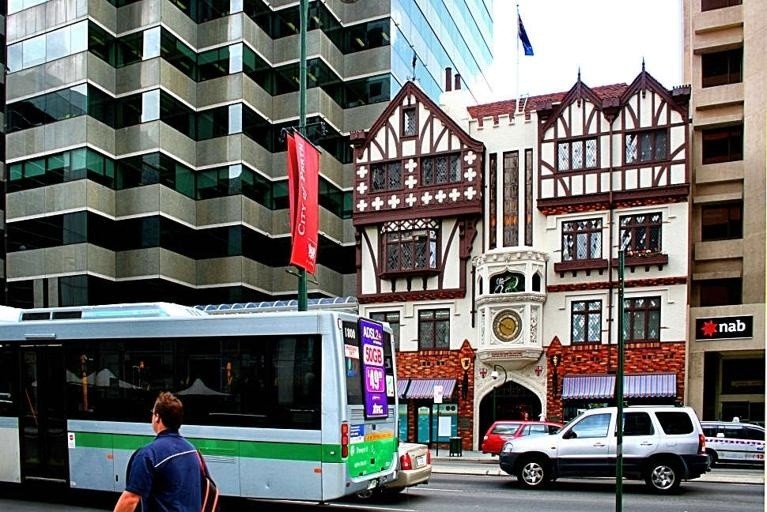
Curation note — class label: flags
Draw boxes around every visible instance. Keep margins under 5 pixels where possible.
[518,15,532,56]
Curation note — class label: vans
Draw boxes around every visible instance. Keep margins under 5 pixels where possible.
[481,405,765,495]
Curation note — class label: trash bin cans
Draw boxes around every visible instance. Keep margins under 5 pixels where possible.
[449,436,463,457]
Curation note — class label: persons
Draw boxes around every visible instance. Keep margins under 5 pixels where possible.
[112,391,220,511]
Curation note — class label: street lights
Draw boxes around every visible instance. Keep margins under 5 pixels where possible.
[434,386,443,456]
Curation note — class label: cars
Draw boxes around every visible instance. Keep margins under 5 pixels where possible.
[355,438,432,500]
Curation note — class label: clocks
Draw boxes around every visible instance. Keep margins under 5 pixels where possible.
[491,307,522,344]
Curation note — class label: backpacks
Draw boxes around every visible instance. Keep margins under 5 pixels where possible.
[190,448,220,512]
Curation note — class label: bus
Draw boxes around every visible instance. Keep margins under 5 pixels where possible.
[0,302,399,502]
